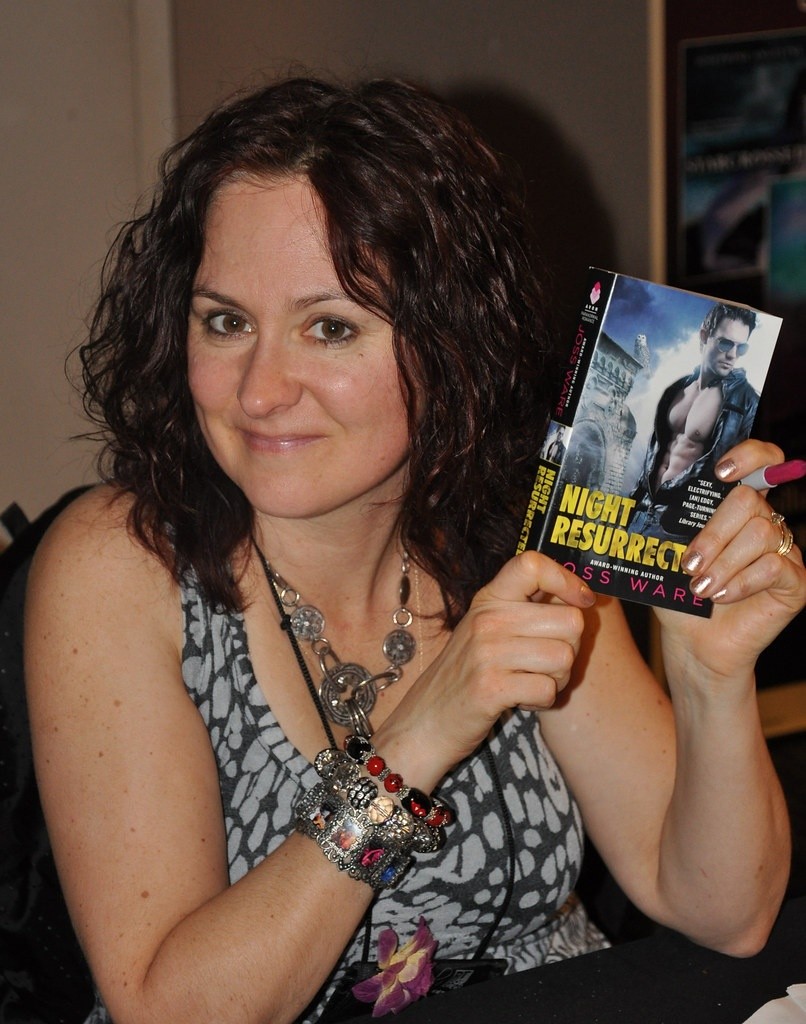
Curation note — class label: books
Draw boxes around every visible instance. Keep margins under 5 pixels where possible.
[516,266,783,618]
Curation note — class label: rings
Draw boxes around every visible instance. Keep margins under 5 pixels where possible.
[770,512,793,555]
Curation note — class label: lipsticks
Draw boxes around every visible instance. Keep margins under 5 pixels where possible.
[739,460,806,491]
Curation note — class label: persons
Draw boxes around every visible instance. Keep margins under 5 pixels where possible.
[0,79,806,1024]
[546,425,566,465]
[597,302,760,571]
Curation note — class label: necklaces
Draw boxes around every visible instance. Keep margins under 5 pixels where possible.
[250,537,424,752]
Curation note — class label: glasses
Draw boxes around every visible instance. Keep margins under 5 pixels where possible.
[710,334,749,356]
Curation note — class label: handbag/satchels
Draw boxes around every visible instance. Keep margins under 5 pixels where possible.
[314,959,508,1024]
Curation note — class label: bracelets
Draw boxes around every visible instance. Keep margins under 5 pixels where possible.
[342,735,452,827]
[314,748,442,852]
[294,783,410,889]
